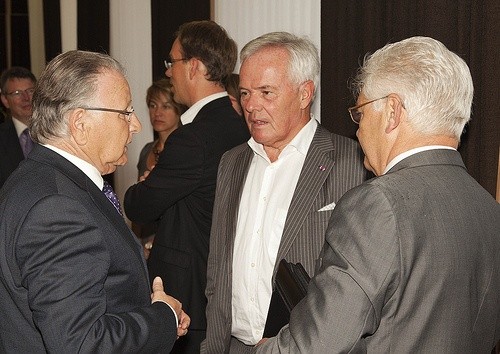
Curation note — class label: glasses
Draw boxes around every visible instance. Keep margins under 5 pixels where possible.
[69,107,134,123]
[3,88,35,97]
[164,57,205,69]
[348,96,406,123]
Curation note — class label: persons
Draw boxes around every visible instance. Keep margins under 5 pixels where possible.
[0,51,191,354]
[0,65,38,189]
[122,20,250,354]
[201,30,372,354]
[253,35,500,354]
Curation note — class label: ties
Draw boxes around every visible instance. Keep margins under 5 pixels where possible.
[24,129,32,156]
[103,179,124,218]
